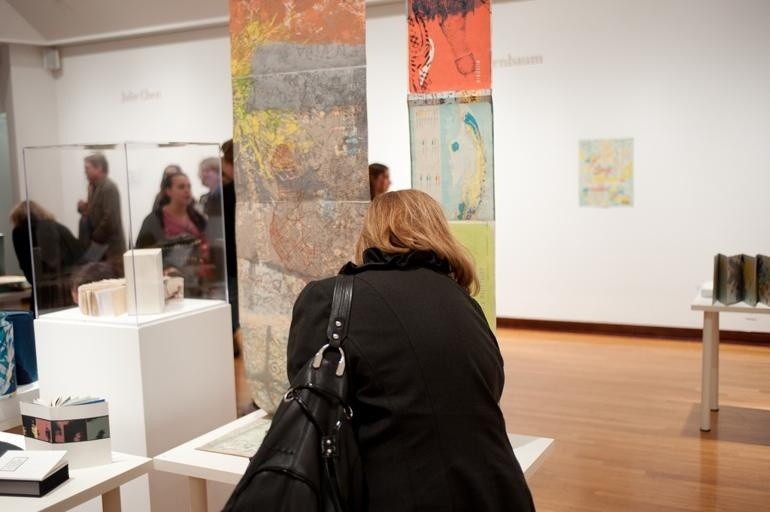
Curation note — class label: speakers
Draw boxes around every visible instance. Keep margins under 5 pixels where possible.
[43,48,60,72]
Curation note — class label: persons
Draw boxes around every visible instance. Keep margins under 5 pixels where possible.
[369,164,391,201]
[133,139,244,361]
[288,190,536,512]
[9,200,79,310]
[78,155,126,279]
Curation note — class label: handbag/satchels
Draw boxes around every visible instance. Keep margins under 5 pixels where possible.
[221,345,360,512]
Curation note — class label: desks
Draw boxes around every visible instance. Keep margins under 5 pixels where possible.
[0,428,157,512]
[150,403,558,511]
[687,278,769,434]
